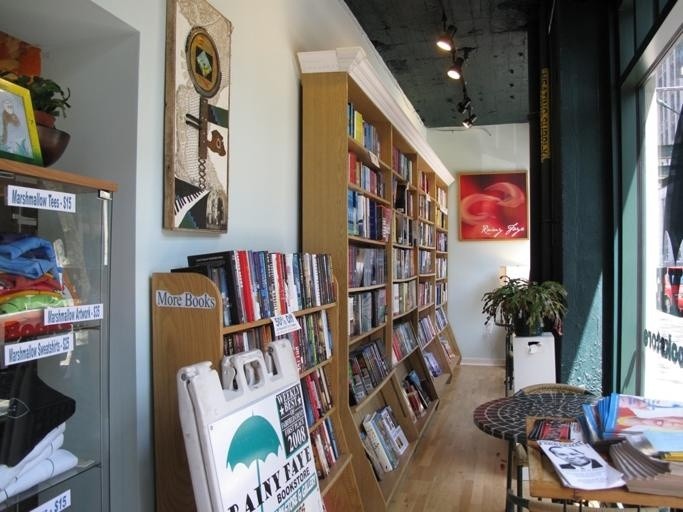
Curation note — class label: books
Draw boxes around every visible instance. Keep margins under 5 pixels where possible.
[577,391,683,447]
[348,151,385,199]
[393,247,414,279]
[436,254,448,278]
[437,209,446,229]
[309,416,340,479]
[436,184,446,209]
[348,337,392,406]
[392,175,413,218]
[420,194,432,221]
[393,369,433,424]
[391,318,418,365]
[418,314,436,346]
[436,281,447,305]
[608,428,683,498]
[528,418,583,443]
[349,245,387,288]
[419,249,432,274]
[419,171,431,195]
[393,280,416,316]
[419,281,433,307]
[359,406,410,482]
[348,101,382,160]
[223,310,333,389]
[393,211,417,247]
[349,189,392,243]
[435,306,448,333]
[392,144,412,184]
[422,347,443,377]
[348,288,386,336]
[439,336,456,362]
[170,250,336,327]
[300,366,333,428]
[436,231,447,252]
[419,221,432,247]
[537,440,626,490]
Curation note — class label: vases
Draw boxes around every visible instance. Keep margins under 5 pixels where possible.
[32,110,72,168]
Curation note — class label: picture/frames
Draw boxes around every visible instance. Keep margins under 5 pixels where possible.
[458,171,530,239]
[0,79,42,167]
[164,0,232,236]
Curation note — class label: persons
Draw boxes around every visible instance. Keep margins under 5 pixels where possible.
[549,446,602,469]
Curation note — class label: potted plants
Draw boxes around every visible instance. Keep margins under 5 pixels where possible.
[481,275,569,336]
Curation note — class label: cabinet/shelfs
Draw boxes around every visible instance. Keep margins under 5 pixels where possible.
[511,333,556,394]
[0,159,119,512]
[296,72,462,512]
[151,267,363,512]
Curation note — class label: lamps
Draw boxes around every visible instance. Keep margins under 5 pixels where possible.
[463,116,479,130]
[436,24,457,52]
[448,56,462,82]
[456,98,470,113]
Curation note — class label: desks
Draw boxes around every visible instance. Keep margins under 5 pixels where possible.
[473,394,600,512]
[524,414,683,512]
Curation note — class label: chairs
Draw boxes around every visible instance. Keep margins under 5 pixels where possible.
[515,383,598,508]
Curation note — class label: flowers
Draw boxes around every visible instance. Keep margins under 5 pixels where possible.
[1,69,72,120]
